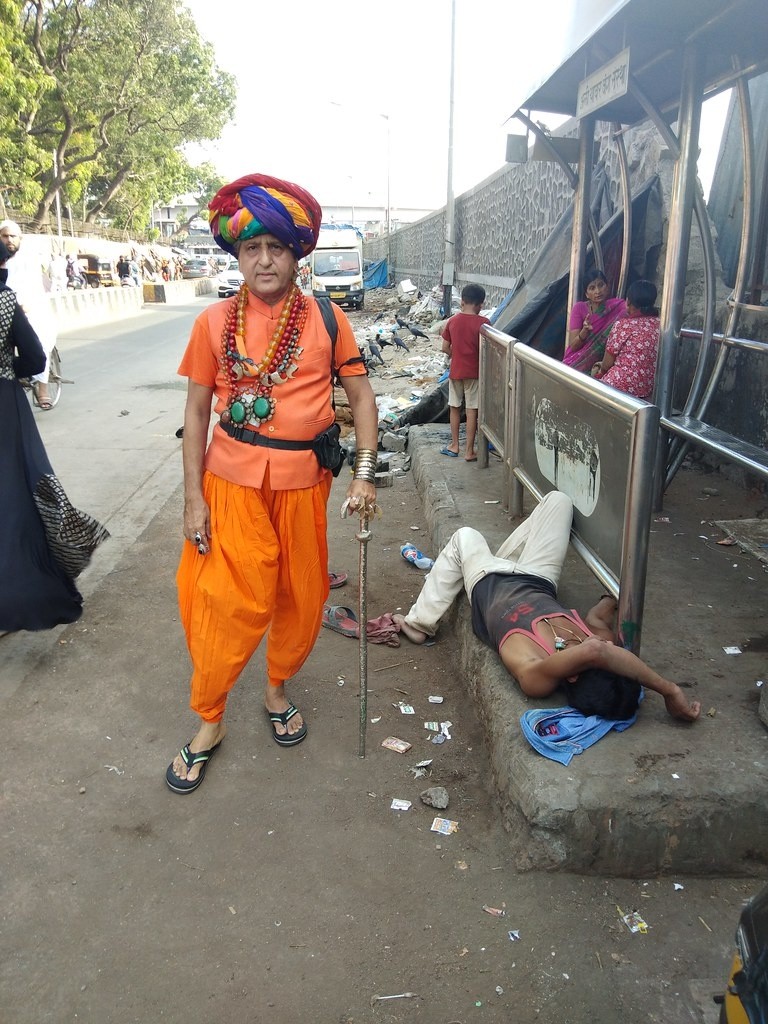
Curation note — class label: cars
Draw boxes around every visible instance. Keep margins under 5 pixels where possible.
[217,261,245,298]
[183,260,216,279]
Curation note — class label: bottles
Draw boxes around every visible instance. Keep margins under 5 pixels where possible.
[400,542,434,569]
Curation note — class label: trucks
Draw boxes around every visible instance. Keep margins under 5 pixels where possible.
[311,229,365,311]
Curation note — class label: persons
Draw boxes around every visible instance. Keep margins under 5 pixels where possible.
[441,284,492,460]
[0,240,111,639]
[165,175,377,794]
[207,255,220,276]
[0,220,58,410]
[49,248,186,291]
[298,265,310,286]
[391,491,700,723]
[591,281,661,398]
[562,270,626,379]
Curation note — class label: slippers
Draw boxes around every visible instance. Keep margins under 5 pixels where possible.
[441,445,458,457]
[329,571,348,590]
[466,457,477,462]
[268,696,307,747]
[165,735,222,795]
[321,603,359,639]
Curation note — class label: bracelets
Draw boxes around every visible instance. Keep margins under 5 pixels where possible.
[578,334,587,341]
[354,449,377,484]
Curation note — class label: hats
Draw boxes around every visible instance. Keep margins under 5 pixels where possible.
[209,174,322,260]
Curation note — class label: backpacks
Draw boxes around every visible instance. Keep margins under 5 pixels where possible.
[66,261,75,278]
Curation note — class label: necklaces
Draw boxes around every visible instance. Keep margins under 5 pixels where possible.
[220,282,309,428]
[545,617,583,653]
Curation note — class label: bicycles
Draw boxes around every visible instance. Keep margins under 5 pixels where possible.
[300,270,307,289]
[17,347,74,410]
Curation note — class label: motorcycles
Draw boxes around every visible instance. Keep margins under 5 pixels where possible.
[75,254,113,287]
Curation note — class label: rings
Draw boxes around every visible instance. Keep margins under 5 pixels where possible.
[352,497,374,510]
[198,546,210,555]
[195,534,208,544]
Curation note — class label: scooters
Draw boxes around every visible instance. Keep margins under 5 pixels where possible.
[66,267,87,291]
[121,274,136,289]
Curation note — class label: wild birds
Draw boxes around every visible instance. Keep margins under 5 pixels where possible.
[366,339,382,361]
[374,309,388,323]
[408,325,428,339]
[376,334,392,350]
[395,315,407,329]
[390,328,409,352]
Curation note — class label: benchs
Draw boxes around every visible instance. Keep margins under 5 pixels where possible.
[651,416,768,515]
